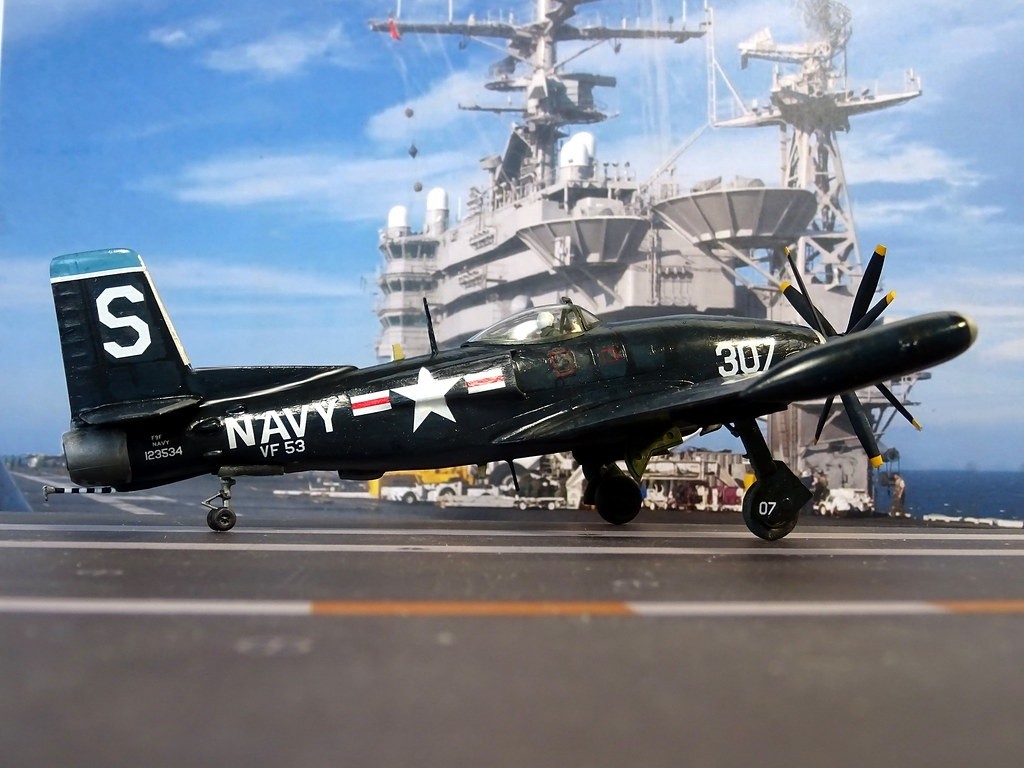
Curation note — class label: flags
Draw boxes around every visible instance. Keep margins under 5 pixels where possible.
[389,20,400,41]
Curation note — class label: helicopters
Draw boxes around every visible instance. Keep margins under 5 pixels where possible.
[43,243,979,541]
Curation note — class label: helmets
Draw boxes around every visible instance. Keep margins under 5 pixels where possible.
[536,311,555,330]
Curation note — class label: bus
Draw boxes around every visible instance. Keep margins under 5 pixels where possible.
[381,469,463,506]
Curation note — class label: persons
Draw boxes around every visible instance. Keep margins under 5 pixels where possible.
[890,473,905,517]
[812,471,828,503]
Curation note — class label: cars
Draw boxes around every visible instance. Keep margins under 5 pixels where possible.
[512,483,875,520]
